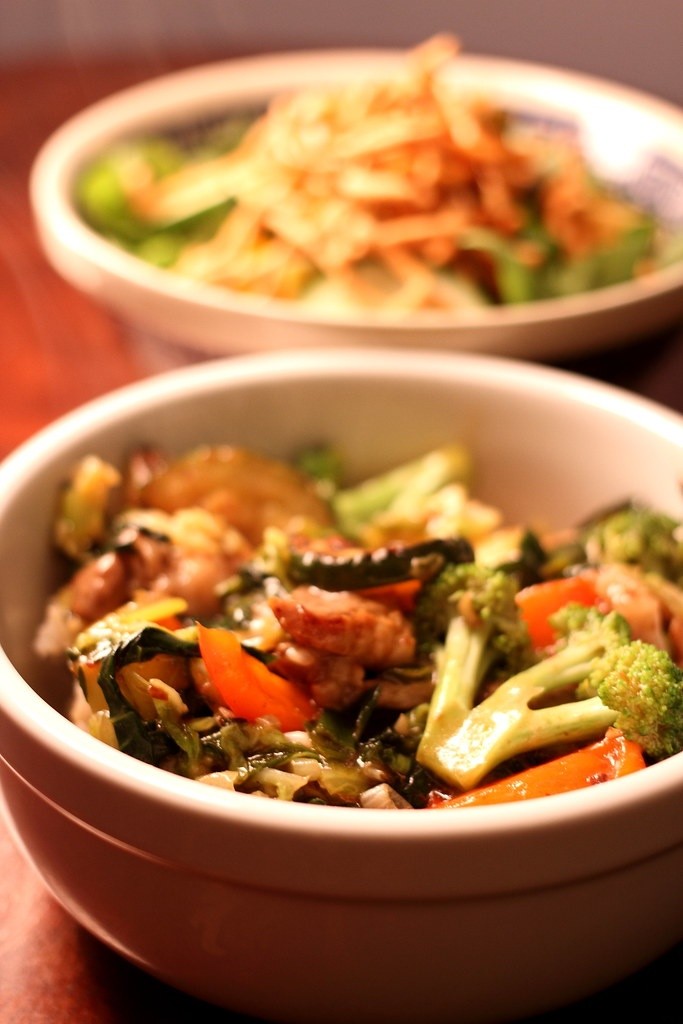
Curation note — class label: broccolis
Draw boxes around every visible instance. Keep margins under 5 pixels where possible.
[295,435,683,787]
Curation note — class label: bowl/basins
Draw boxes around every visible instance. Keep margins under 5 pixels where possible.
[0,347,683,1015]
[29,46,683,385]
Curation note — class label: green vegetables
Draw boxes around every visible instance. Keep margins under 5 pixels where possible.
[70,113,652,312]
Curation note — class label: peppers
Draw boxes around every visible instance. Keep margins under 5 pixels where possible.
[192,534,683,810]
